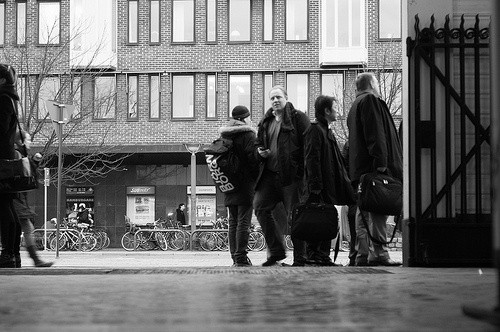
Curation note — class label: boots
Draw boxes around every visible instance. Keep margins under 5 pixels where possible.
[26,245,53,267]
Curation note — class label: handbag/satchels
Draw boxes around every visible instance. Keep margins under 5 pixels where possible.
[291,191,339,241]
[0,156,39,192]
[355,167,403,215]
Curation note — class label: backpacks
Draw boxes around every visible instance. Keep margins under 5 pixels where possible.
[206,132,252,195]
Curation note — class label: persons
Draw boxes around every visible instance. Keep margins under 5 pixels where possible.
[76,203,93,230]
[0,63,58,268]
[218,106,257,267]
[252,72,403,268]
[176,203,186,225]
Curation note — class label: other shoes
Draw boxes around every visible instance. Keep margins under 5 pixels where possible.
[234,261,253,267]
[356,261,402,266]
[263,255,286,267]
[292,261,343,267]
[0,253,21,268]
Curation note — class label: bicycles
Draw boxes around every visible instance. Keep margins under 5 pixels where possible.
[35,213,111,252]
[120,216,230,252]
[340,240,352,251]
[285,235,295,250]
[246,224,267,252]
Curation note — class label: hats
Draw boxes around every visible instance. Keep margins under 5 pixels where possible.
[232,106,251,121]
